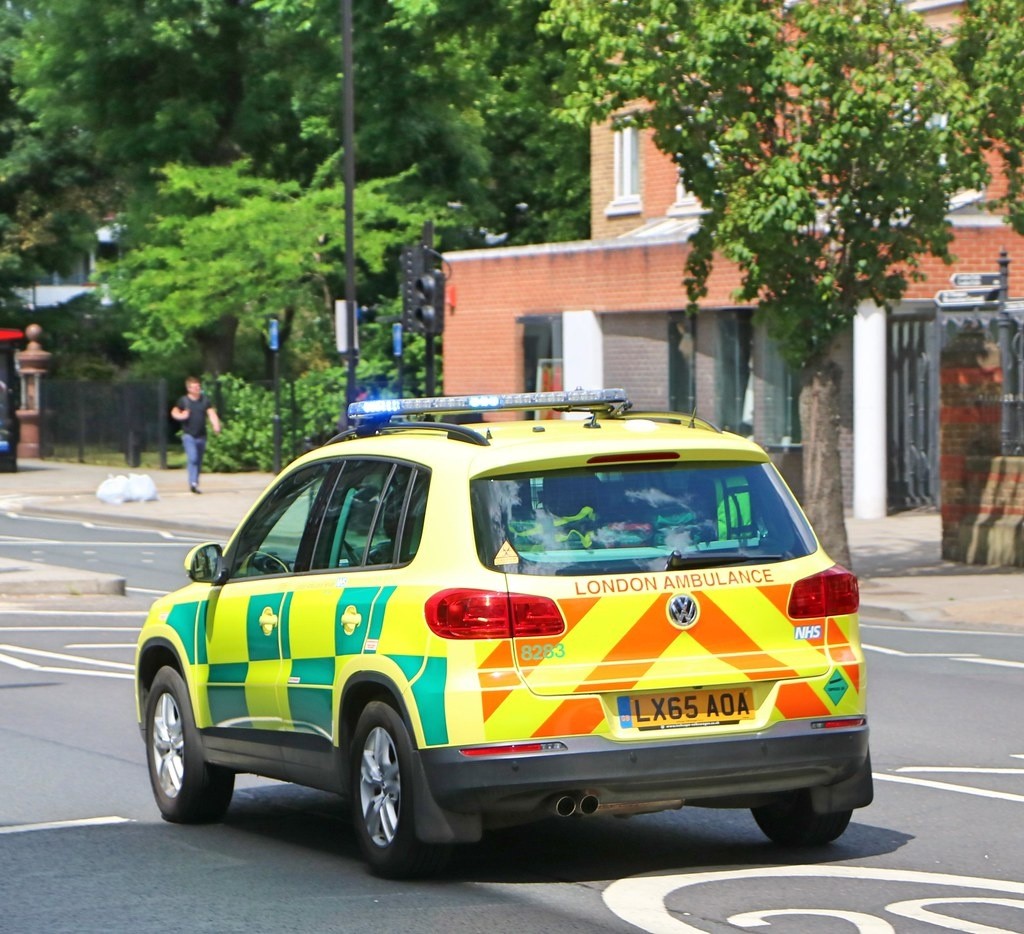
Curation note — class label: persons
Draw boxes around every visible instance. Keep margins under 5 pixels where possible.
[171,374,223,494]
[360,487,414,566]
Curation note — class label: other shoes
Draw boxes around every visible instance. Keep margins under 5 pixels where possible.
[191,482,201,494]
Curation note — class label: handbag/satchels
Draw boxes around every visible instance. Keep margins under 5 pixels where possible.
[174,429,183,443]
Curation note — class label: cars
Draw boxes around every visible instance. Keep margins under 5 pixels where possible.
[133,385,874,882]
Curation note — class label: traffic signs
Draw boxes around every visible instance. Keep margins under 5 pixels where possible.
[948,271,1003,287]
[934,285,1001,308]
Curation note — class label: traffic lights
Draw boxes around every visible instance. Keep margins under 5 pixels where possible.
[409,264,445,336]
